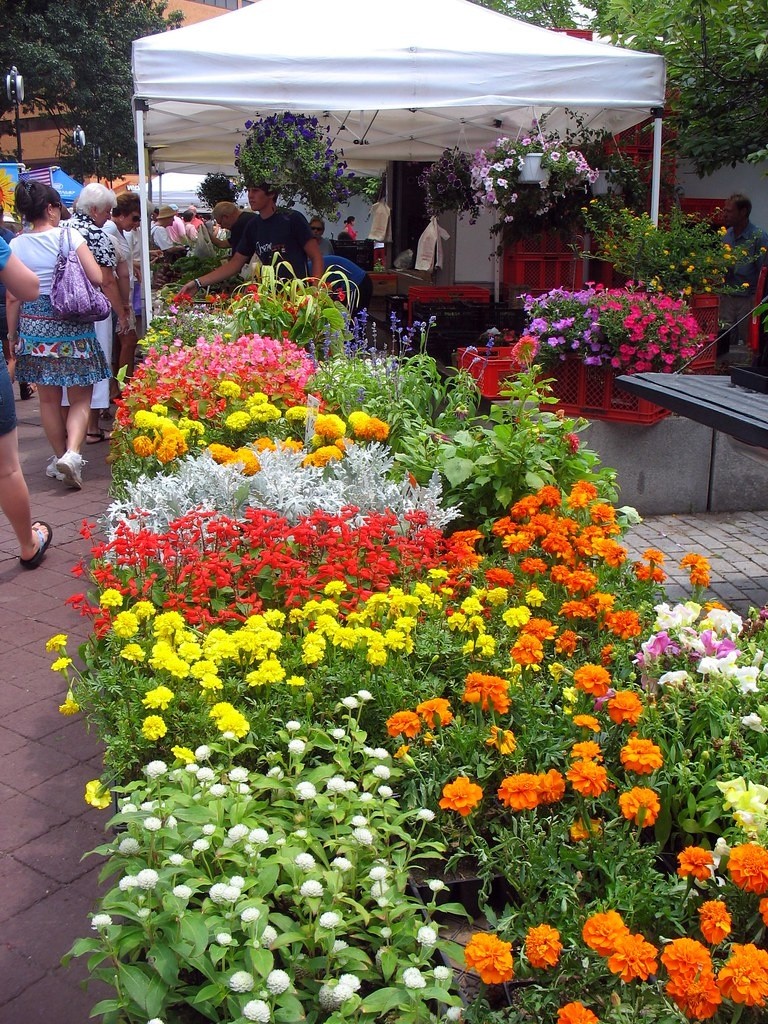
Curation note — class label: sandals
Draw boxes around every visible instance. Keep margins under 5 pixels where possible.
[100,408,112,420]
[86,428,114,444]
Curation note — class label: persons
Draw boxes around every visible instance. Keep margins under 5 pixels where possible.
[58,182,142,444]
[721,194,768,344]
[0,235,53,569]
[179,182,323,298]
[344,216,358,240]
[308,256,373,352]
[0,206,37,400]
[308,215,334,256]
[6,180,112,491]
[150,201,259,279]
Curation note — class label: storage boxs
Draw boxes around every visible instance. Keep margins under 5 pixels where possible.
[602,263,642,289]
[552,29,683,185]
[501,283,555,310]
[669,198,725,224]
[503,230,585,260]
[408,284,488,332]
[385,294,409,325]
[412,302,488,339]
[677,295,718,368]
[492,306,531,335]
[625,190,672,228]
[501,256,583,292]
[458,347,524,397]
[328,237,370,269]
[533,346,673,425]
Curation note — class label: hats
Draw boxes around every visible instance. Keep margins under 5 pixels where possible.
[156,205,178,218]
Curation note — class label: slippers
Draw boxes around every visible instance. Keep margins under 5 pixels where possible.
[20,520,52,567]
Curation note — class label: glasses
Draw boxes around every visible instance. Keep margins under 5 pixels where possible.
[48,200,62,208]
[129,211,141,222]
[311,226,323,231]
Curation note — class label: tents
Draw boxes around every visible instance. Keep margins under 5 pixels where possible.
[136,171,260,214]
[131,0,667,330]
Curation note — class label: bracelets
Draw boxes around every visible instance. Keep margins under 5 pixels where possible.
[7,331,19,340]
[195,279,201,290]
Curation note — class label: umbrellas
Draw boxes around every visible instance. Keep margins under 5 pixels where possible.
[52,167,83,207]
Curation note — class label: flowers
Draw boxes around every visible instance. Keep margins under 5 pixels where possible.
[414,116,607,264]
[195,170,246,212]
[231,109,382,224]
[44,201,768,1024]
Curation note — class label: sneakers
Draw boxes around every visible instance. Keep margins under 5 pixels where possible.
[47,455,66,480]
[56,450,88,489]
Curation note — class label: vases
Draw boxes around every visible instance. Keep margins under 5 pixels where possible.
[515,149,551,186]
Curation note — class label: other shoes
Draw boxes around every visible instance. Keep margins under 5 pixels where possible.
[20,382,37,400]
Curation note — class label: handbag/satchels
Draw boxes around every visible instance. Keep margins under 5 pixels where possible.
[195,224,217,258]
[50,227,112,321]
